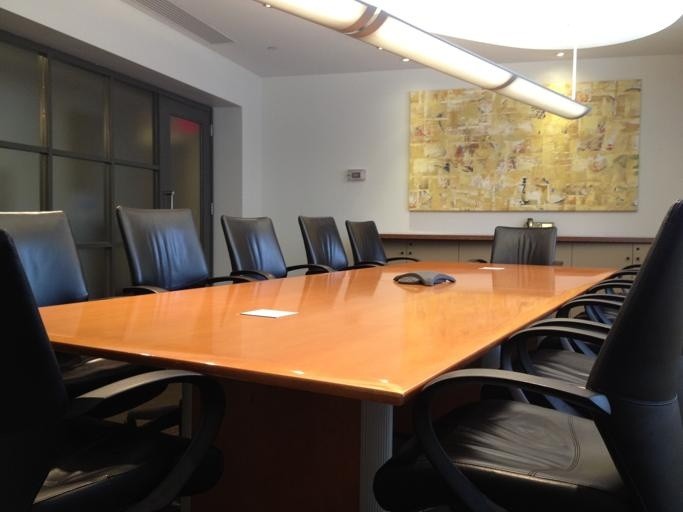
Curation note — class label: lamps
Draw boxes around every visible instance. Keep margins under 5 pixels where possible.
[253,0,591,120]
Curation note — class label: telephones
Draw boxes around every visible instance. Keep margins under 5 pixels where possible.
[393,271,456,286]
